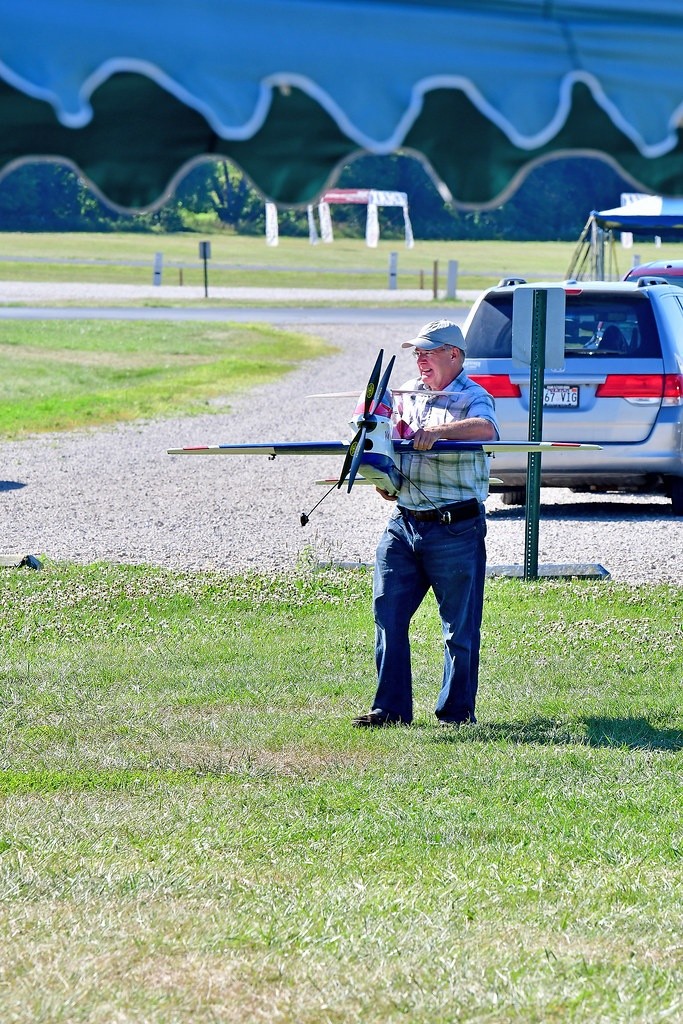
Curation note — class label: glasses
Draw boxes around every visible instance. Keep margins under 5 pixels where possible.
[412,348,445,358]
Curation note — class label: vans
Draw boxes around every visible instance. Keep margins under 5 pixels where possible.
[592,258,683,345]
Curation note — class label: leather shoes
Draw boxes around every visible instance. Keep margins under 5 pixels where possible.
[352,708,397,728]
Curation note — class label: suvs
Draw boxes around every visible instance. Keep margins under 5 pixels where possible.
[459,275,683,516]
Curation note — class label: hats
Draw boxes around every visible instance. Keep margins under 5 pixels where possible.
[402,319,467,351]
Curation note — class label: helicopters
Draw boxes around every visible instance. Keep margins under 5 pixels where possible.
[164,347,603,524]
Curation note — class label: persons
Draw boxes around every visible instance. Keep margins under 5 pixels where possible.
[351,318,501,725]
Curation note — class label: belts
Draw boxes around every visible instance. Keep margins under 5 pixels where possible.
[407,501,480,522]
